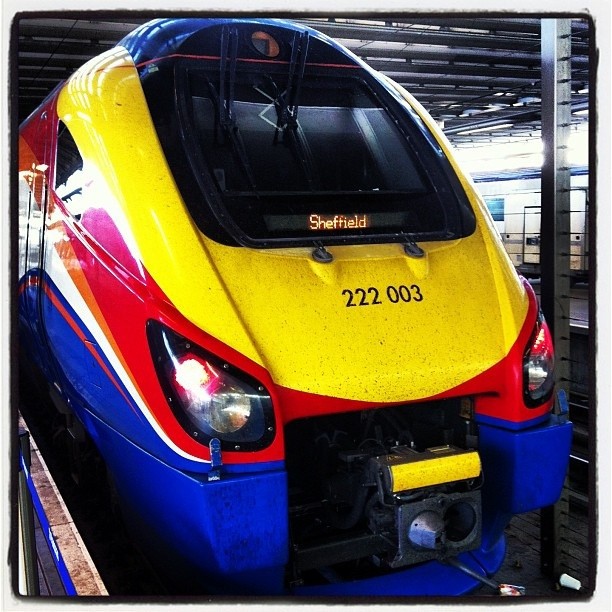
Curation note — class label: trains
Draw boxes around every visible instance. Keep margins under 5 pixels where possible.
[474,175,590,286]
[16,18,573,595]
